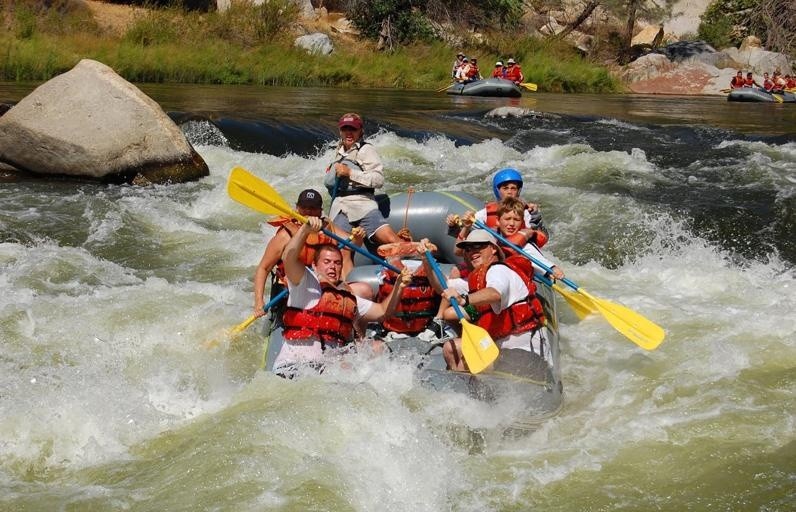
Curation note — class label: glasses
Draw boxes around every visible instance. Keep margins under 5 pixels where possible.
[464,242,484,252]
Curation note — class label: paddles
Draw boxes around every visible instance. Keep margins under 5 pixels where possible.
[423,247,500,375]
[469,216,666,351]
[719,88,730,92]
[454,215,597,320]
[519,82,538,92]
[760,86,784,103]
[227,166,406,276]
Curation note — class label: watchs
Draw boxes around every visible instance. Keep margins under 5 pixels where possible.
[460,294,469,307]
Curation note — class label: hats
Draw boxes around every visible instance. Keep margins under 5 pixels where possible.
[298,189,322,209]
[495,61,502,65]
[463,58,468,62]
[456,229,505,261]
[507,59,515,63]
[470,56,477,59]
[338,113,362,129]
[456,52,465,58]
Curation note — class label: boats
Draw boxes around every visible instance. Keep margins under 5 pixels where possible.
[446,79,522,96]
[727,87,795,102]
[263,190,565,455]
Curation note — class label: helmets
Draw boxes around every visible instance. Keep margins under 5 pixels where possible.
[493,168,523,200]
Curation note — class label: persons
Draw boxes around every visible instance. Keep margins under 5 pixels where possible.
[449,195,567,284]
[322,111,411,257]
[452,51,523,84]
[253,188,374,339]
[415,227,555,396]
[267,215,414,382]
[445,169,550,238]
[373,227,457,345]
[731,69,796,94]
